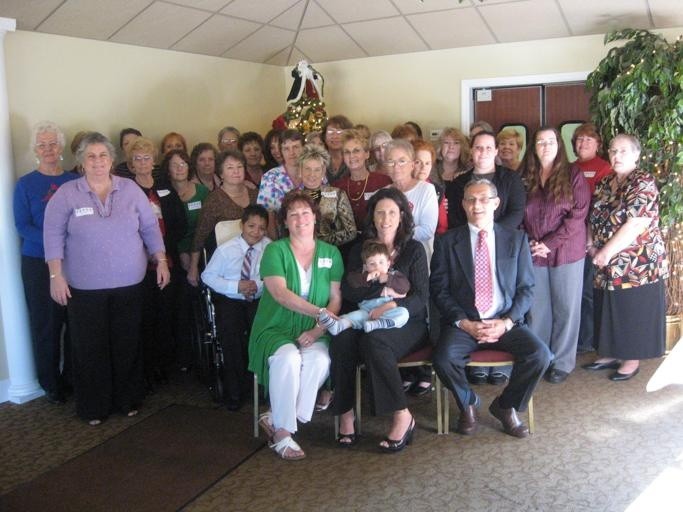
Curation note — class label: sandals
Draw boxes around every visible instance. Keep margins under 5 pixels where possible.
[315,390,333,413]
[84,415,104,426]
[257,410,307,460]
[119,401,138,417]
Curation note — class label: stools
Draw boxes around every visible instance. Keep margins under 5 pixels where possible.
[248,344,342,447]
[352,342,443,440]
[438,348,536,438]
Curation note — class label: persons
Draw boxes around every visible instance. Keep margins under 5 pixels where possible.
[582,132,669,381]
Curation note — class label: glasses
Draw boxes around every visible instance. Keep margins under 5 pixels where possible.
[34,141,60,150]
[325,128,343,137]
[170,163,188,170]
[462,196,495,206]
[536,138,557,146]
[386,159,412,167]
[133,154,151,163]
[373,144,386,152]
[221,137,238,146]
[607,147,629,155]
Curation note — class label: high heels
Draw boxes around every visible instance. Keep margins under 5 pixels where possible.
[337,409,356,447]
[379,415,417,452]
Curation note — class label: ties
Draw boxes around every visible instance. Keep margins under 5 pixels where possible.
[240,247,255,303]
[474,230,494,315]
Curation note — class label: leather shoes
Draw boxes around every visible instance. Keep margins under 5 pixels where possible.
[459,388,481,434]
[582,358,622,371]
[610,368,639,380]
[472,373,507,385]
[45,390,65,406]
[402,371,436,396]
[548,367,569,383]
[488,396,529,438]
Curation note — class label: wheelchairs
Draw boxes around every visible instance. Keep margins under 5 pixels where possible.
[196,218,243,409]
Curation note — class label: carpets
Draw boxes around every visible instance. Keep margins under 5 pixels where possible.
[0,402,270,512]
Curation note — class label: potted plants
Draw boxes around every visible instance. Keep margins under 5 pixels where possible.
[578,26,682,356]
